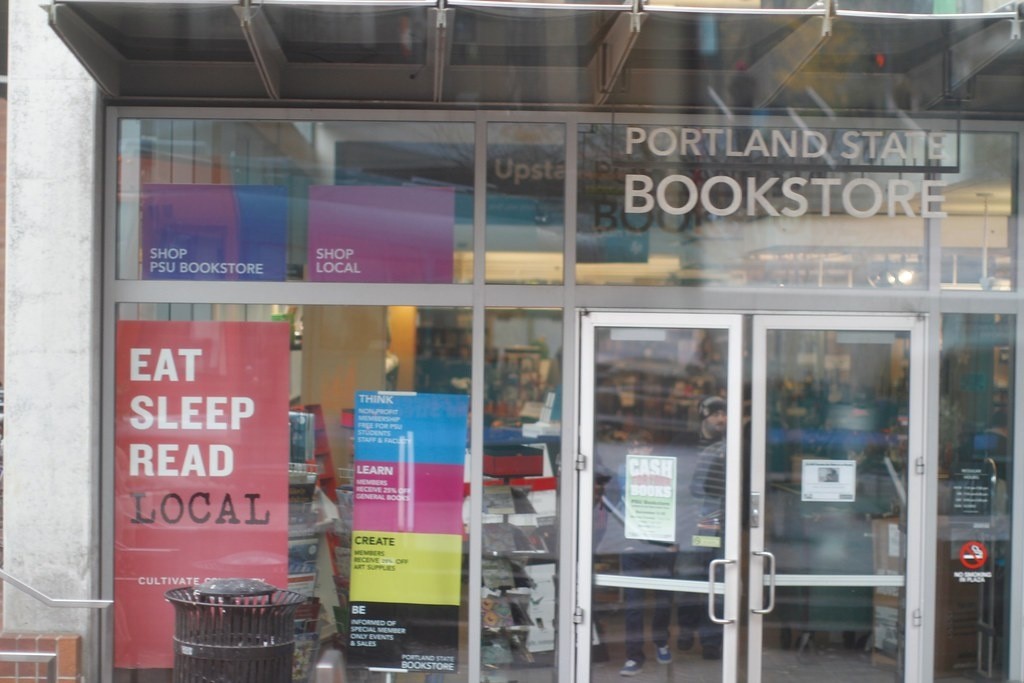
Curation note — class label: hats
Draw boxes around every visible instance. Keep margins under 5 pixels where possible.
[697,396,727,422]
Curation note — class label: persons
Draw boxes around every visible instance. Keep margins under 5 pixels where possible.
[960,412,1009,481]
[668,395,727,661]
[773,420,900,652]
[603,431,683,676]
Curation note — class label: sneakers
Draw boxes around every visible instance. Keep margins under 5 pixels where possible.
[620,660,643,676]
[654,645,673,664]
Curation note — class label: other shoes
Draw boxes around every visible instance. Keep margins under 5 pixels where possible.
[842,629,857,650]
[703,645,721,660]
[781,625,792,650]
[852,631,871,655]
[676,627,694,651]
[802,630,815,651]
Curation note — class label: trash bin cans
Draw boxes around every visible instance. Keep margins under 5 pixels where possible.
[163,580,308,683]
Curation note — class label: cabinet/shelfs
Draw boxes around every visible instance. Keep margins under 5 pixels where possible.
[482,484,550,675]
[288,405,328,679]
[872,517,993,672]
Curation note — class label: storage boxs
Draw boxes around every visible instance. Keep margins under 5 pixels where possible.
[485,445,546,477]
